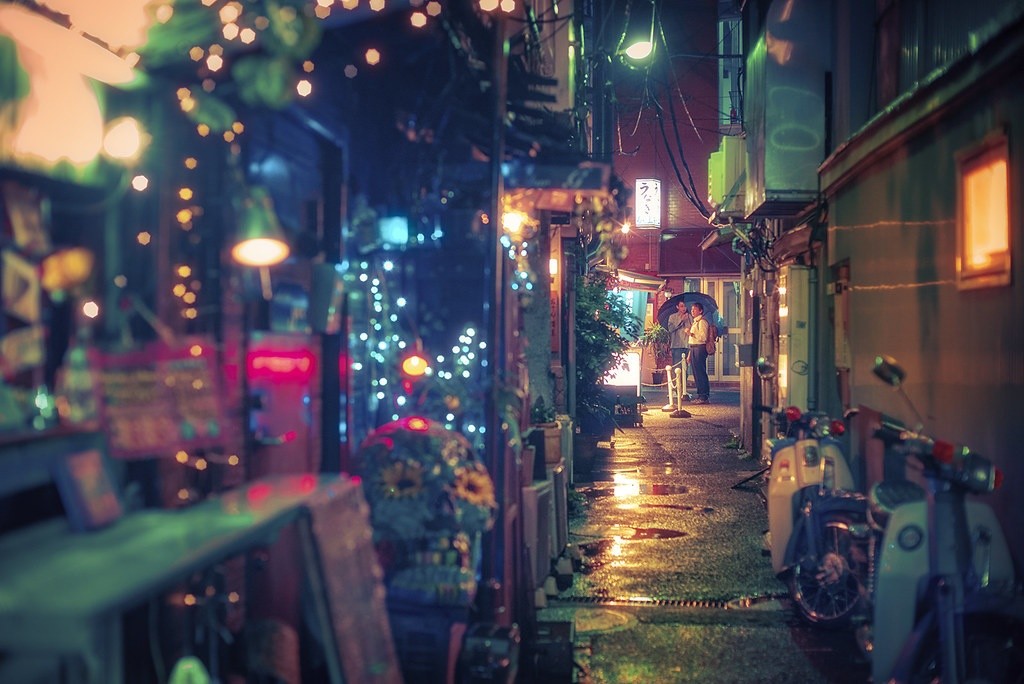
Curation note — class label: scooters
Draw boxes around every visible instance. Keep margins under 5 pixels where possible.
[754,353,1024,684]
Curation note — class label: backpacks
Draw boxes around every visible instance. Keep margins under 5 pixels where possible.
[697,318,718,354]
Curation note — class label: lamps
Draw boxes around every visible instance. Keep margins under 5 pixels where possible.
[596,30,653,58]
[225,184,288,269]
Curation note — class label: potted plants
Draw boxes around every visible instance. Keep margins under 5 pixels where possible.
[530,394,562,463]
[644,320,670,387]
[574,279,641,474]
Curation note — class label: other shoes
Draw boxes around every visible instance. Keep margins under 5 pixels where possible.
[693,398,709,405]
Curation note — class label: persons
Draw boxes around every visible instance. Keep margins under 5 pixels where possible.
[668,301,693,394]
[683,303,710,405]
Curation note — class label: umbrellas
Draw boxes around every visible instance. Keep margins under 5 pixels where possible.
[656,291,718,331]
